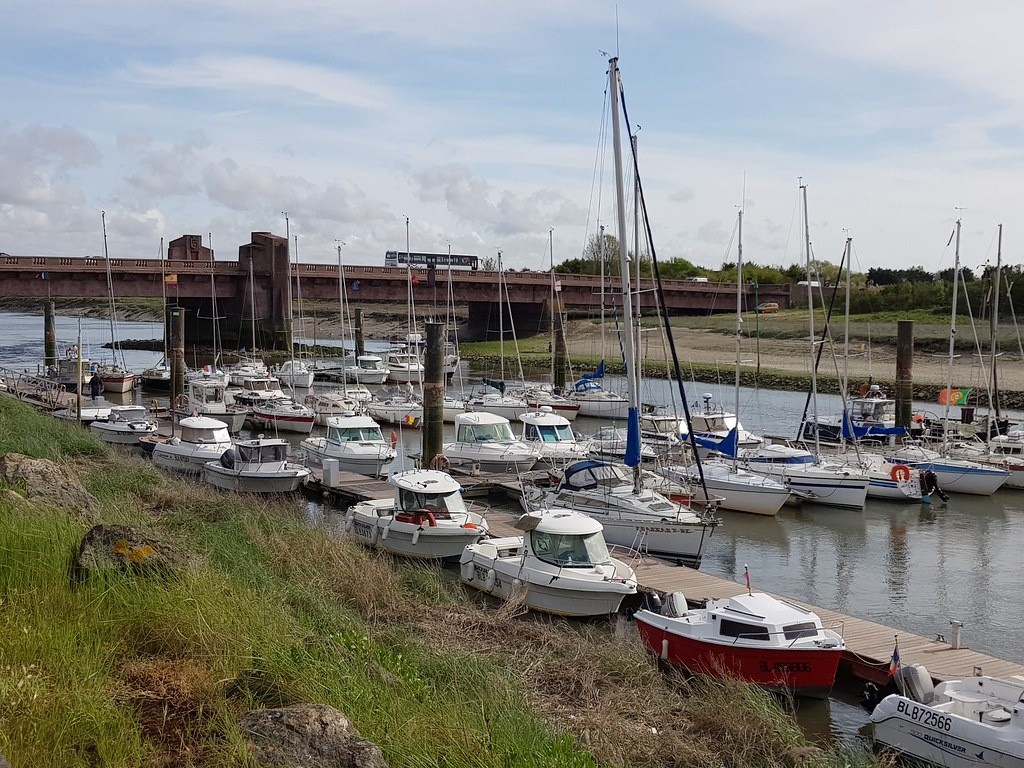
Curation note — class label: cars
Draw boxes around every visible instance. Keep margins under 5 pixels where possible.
[754,302,779,314]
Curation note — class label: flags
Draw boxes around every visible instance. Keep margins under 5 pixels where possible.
[165,273,177,284]
[939,388,973,405]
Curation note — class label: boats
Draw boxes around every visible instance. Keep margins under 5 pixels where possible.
[630,563,848,700]
[856,632,1024,768]
[459,456,649,623]
[342,379,490,564]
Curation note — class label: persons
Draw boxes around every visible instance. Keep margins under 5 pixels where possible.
[67,345,78,361]
[88,372,103,401]
[88,359,98,376]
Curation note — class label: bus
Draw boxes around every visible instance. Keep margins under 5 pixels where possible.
[384,249,483,275]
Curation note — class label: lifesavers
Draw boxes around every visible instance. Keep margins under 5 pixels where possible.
[892,465,910,482]
[428,455,451,473]
[416,509,438,526]
[390,431,398,449]
[859,383,871,397]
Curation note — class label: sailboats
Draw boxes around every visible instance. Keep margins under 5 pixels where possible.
[48,1,1023,572]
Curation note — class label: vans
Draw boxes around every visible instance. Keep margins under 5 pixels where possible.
[798,281,820,287]
[683,276,708,286]
